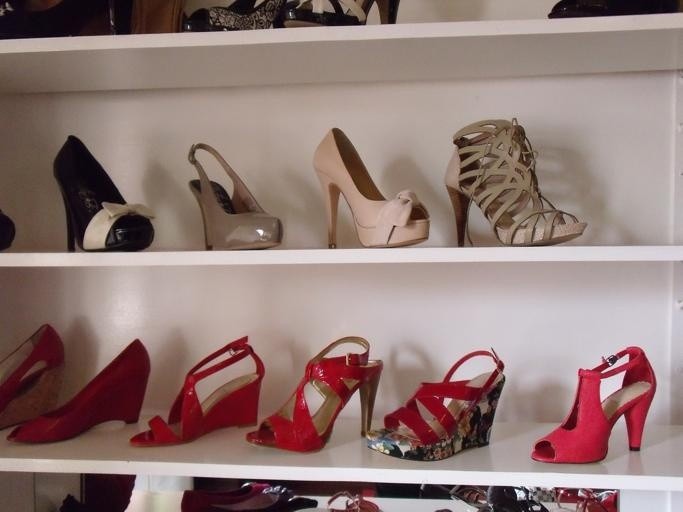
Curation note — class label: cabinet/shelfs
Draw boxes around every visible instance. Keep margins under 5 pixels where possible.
[0,0,682,511]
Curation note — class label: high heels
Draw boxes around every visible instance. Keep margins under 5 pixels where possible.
[132,337,265,447]
[50,136,157,251]
[2,321,148,443]
[534,347,657,464]
[184,0,399,41]
[249,337,384,453]
[3,1,119,35]
[365,350,505,460]
[186,143,282,250]
[312,127,431,246]
[446,119,588,248]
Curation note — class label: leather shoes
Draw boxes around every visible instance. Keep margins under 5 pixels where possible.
[180,475,619,511]
